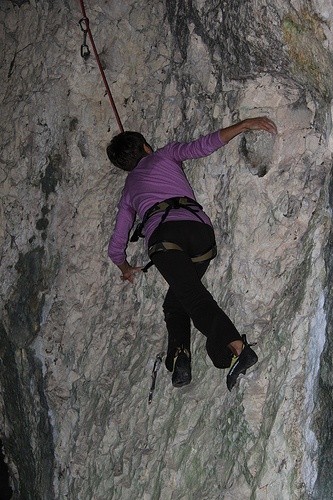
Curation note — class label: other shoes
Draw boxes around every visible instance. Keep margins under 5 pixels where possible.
[171,348,193,387]
[226,345,257,390]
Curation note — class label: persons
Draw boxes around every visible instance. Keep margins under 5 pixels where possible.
[104,115,278,391]
